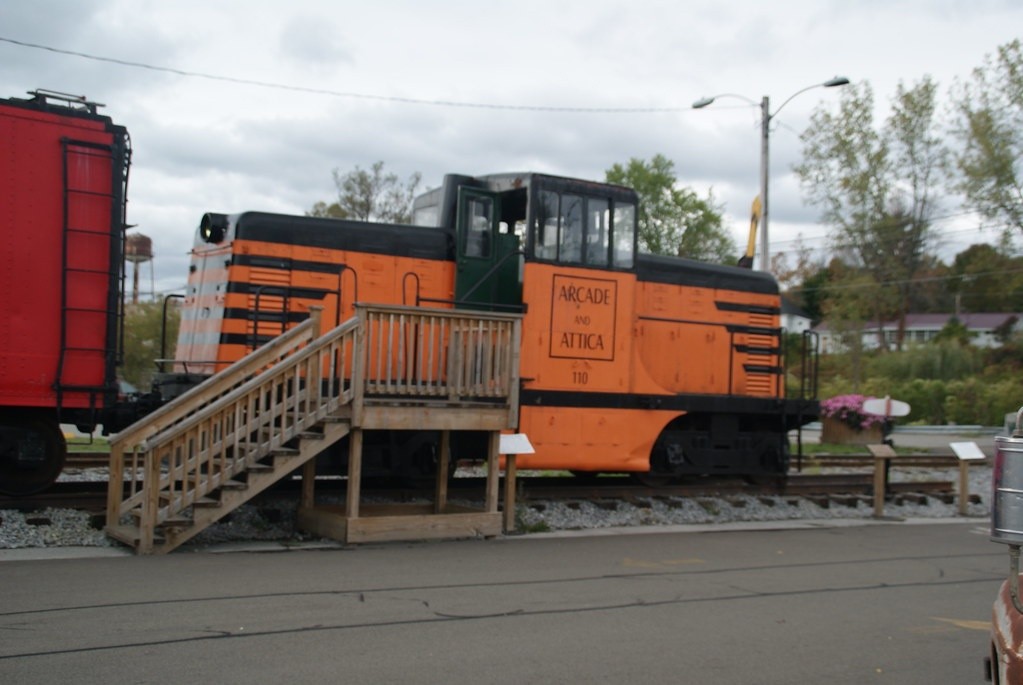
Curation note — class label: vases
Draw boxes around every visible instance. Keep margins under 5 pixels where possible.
[821,417,884,445]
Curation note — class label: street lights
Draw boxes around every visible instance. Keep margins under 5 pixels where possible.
[692,75,849,272]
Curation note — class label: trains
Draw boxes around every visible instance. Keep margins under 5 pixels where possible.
[0,88,822,507]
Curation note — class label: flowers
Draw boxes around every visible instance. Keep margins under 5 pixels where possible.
[820,394,895,430]
